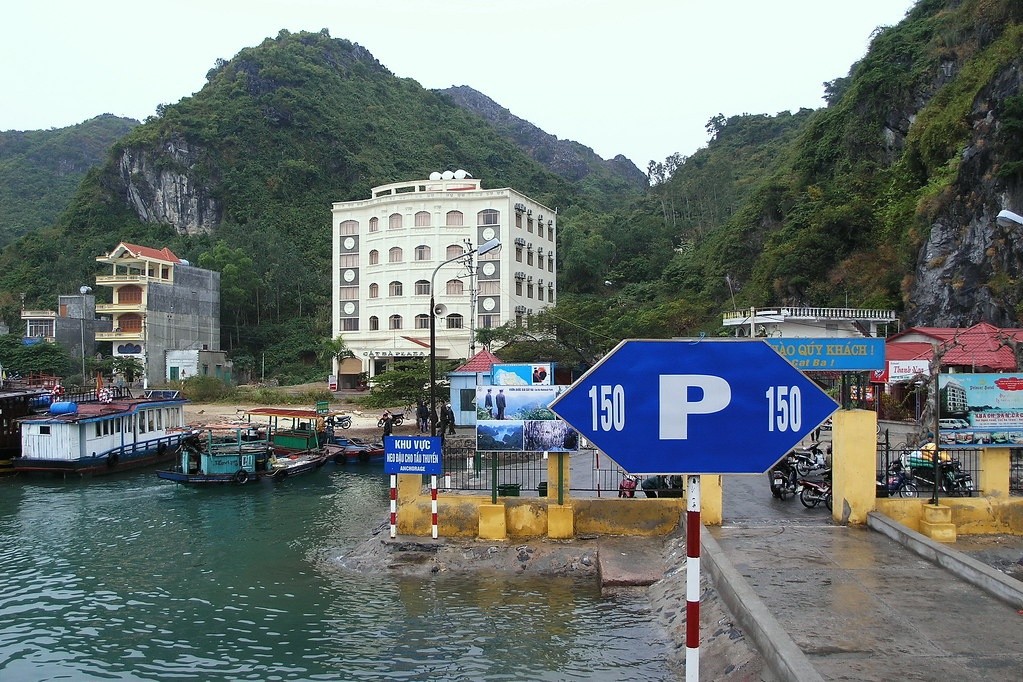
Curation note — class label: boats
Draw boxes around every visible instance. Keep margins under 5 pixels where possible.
[157,408,386,487]
[0,386,204,477]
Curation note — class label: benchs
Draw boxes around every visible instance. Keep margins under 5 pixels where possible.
[273,430,328,454]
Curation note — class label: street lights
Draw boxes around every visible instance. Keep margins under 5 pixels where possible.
[430,237,503,436]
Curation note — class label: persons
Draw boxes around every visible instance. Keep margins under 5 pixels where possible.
[811,427,821,443]
[485,389,493,418]
[378,414,394,449]
[641,475,683,498]
[917,427,934,449]
[416,400,456,435]
[496,389,506,420]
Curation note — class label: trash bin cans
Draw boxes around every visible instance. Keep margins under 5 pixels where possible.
[498,484,521,496]
[537,482,547,496]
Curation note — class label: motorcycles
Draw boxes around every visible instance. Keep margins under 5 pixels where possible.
[377,408,405,427]
[818,419,833,431]
[618,469,642,498]
[876,449,919,499]
[767,440,833,512]
[936,457,976,497]
[324,414,353,429]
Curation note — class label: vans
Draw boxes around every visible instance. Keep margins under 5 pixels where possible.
[938,418,970,430]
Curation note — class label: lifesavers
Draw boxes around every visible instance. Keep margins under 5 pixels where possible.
[54,384,64,396]
[334,453,346,465]
[99,387,113,404]
[50,390,60,403]
[234,469,250,484]
[275,469,288,482]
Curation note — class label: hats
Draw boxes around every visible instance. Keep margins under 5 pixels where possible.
[447,404,451,407]
[927,432,934,438]
[488,389,492,392]
[499,389,503,392]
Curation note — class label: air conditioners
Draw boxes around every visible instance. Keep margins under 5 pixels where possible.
[515,203,526,213]
[527,209,532,216]
[528,275,532,281]
[538,278,543,284]
[548,281,553,287]
[548,251,553,256]
[527,243,533,248]
[538,215,543,221]
[538,247,542,252]
[515,238,525,246]
[539,310,544,314]
[515,272,526,279]
[515,305,532,314]
[548,220,553,226]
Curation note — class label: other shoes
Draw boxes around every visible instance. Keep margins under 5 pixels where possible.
[816,439,821,443]
